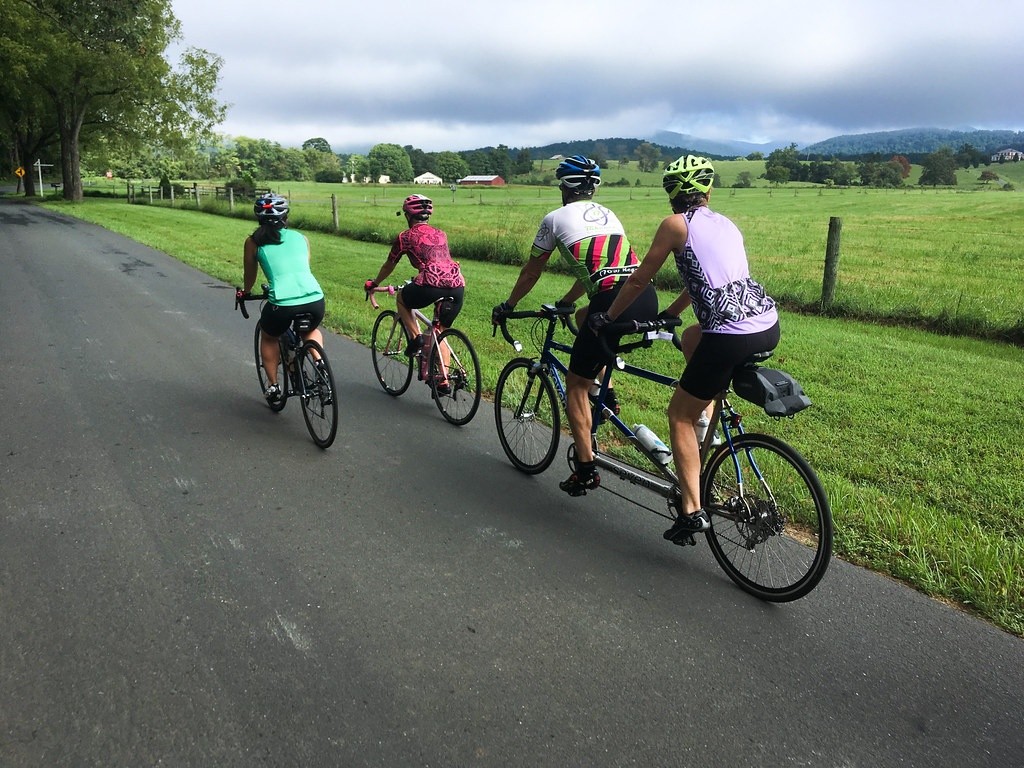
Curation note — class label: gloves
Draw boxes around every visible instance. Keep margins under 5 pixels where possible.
[588,311,609,335]
[236,289,251,301]
[657,310,680,333]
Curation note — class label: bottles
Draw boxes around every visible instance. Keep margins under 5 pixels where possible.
[694,411,710,448]
[588,376,601,395]
[632,421,672,463]
[288,347,295,371]
[422,329,431,356]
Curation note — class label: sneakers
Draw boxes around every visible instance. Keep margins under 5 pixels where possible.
[266,384,281,399]
[663,508,710,540]
[591,397,620,423]
[403,334,424,357]
[559,468,600,492]
[314,359,327,382]
[711,429,722,446]
[431,386,451,399]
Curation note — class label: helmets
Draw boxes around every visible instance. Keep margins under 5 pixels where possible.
[662,155,714,199]
[403,194,433,215]
[555,155,600,189]
[254,193,289,219]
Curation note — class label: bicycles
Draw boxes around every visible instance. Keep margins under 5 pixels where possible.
[235,284,338,448]
[491,306,833,603]
[365,280,482,426]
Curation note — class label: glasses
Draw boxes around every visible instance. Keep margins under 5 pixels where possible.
[559,182,565,190]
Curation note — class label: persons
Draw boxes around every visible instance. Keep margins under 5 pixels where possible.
[364,194,465,399]
[235,193,332,402]
[587,155,781,540]
[491,155,658,491]
